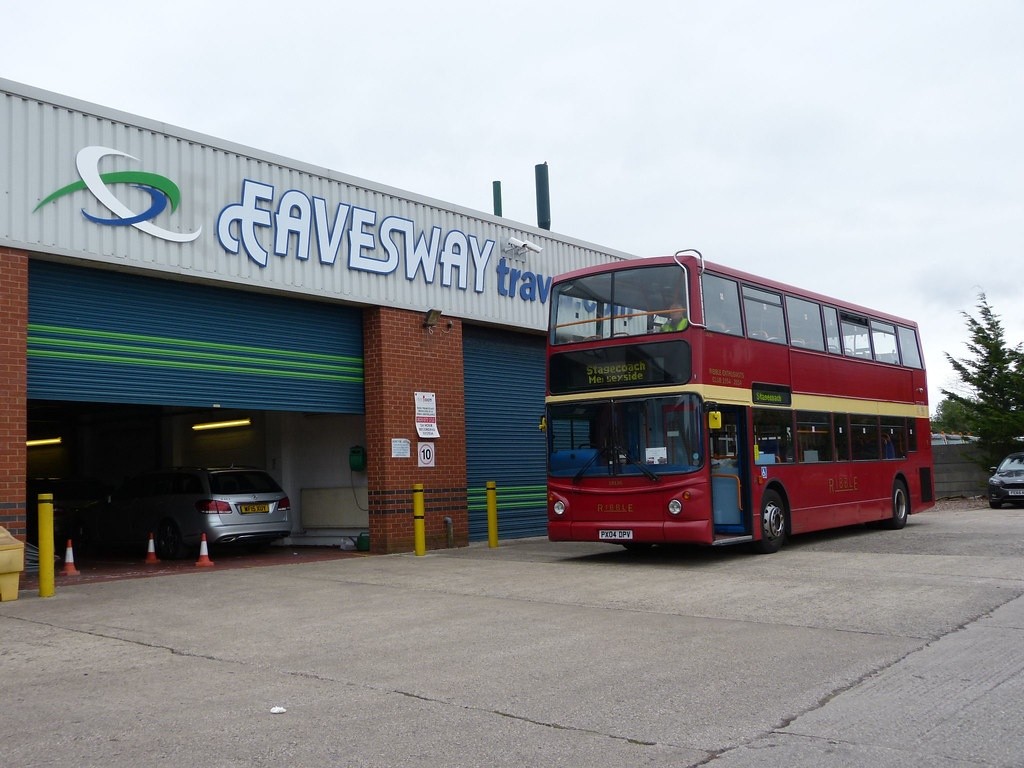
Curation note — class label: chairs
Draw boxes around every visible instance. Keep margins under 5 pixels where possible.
[727,330,894,364]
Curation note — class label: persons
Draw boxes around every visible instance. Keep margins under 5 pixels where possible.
[658,301,688,332]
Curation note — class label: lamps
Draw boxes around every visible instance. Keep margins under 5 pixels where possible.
[25,436,63,447]
[191,417,252,431]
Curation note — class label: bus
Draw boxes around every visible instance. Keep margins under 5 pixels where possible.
[543,251,934,547]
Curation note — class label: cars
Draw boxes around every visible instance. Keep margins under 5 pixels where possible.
[84,464,293,557]
[988,453,1024,509]
